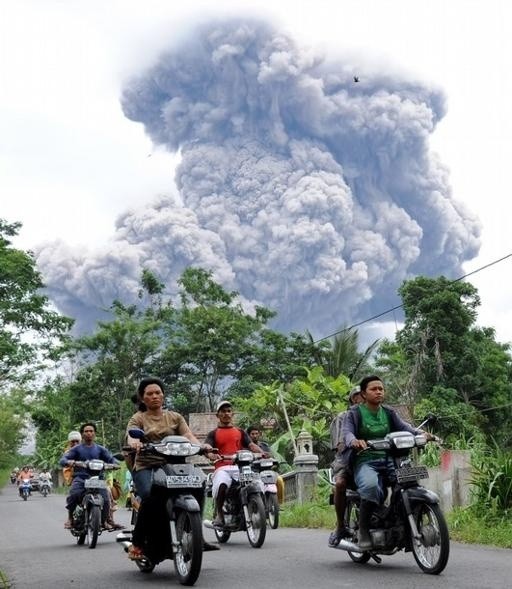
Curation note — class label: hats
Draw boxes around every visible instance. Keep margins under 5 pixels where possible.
[216,401,231,412]
[349,386,361,398]
[68,431,82,441]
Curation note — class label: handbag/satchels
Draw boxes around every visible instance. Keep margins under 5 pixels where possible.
[110,479,122,499]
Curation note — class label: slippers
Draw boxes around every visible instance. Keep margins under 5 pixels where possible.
[111,522,126,531]
[213,519,225,526]
[65,516,74,528]
[327,529,345,546]
[128,545,143,559]
[103,521,112,528]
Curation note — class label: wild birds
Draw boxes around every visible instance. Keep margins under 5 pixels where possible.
[353,75,360,82]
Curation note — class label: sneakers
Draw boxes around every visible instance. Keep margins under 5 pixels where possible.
[357,530,372,547]
[203,543,219,551]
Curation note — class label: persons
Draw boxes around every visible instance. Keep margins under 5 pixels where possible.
[340,374,443,552]
[121,376,214,559]
[124,392,222,554]
[38,466,53,492]
[202,399,272,528]
[246,425,286,512]
[325,383,366,548]
[17,467,35,497]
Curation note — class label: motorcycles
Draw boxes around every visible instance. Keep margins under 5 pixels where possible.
[316,414,449,575]
[65,428,293,585]
[9,476,52,501]
